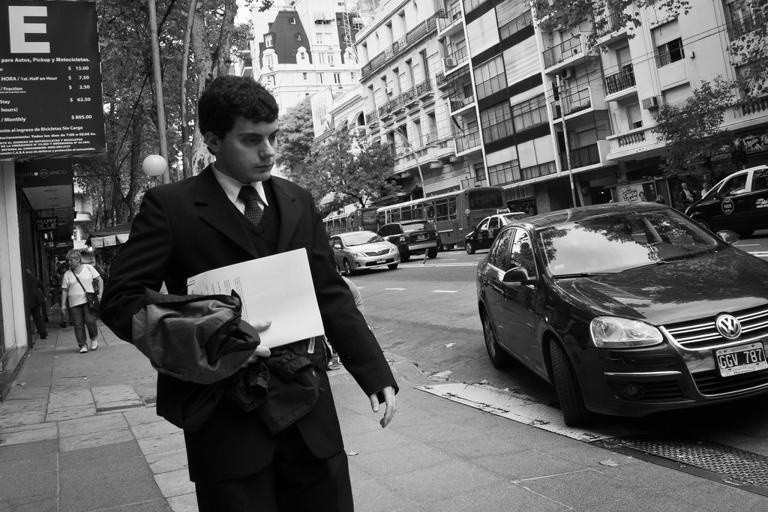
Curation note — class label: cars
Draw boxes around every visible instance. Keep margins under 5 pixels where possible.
[464,212,529,254]
[685,165,768,239]
[477,202,768,427]
[377,220,441,262]
[329,230,401,277]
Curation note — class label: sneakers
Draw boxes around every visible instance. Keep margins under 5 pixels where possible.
[79,343,89,354]
[90,339,98,350]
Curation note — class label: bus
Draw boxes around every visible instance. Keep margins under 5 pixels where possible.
[377,186,508,251]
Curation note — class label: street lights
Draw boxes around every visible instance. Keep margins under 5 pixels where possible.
[143,155,168,188]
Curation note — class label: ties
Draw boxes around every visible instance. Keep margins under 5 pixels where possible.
[237,184,267,230]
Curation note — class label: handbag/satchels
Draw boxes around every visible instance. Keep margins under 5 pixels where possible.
[225,351,334,439]
[85,291,102,312]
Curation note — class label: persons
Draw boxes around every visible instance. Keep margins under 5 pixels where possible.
[98,75,399,512]
[22,263,48,339]
[656,192,664,204]
[681,182,697,210]
[53,261,74,328]
[60,250,104,353]
[639,191,648,202]
[700,182,711,198]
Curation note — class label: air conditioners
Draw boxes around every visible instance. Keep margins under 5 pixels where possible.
[642,97,658,109]
